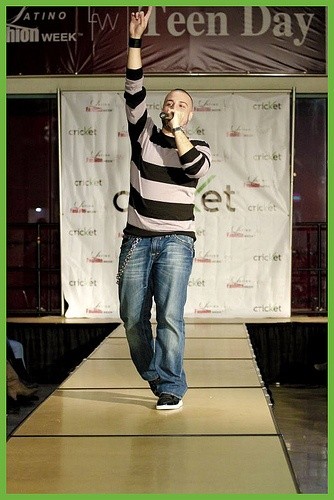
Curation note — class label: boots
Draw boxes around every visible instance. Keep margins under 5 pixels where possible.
[6,360,39,401]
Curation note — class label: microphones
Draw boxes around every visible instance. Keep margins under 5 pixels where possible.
[159,112,173,120]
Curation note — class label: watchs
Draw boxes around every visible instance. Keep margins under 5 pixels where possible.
[172,126,185,136]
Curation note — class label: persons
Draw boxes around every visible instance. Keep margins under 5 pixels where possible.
[116,6,212,410]
[6,337,39,401]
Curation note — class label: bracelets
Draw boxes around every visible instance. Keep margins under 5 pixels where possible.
[128,37,141,48]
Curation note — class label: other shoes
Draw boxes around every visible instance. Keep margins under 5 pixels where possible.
[149,376,163,397]
[155,393,183,410]
